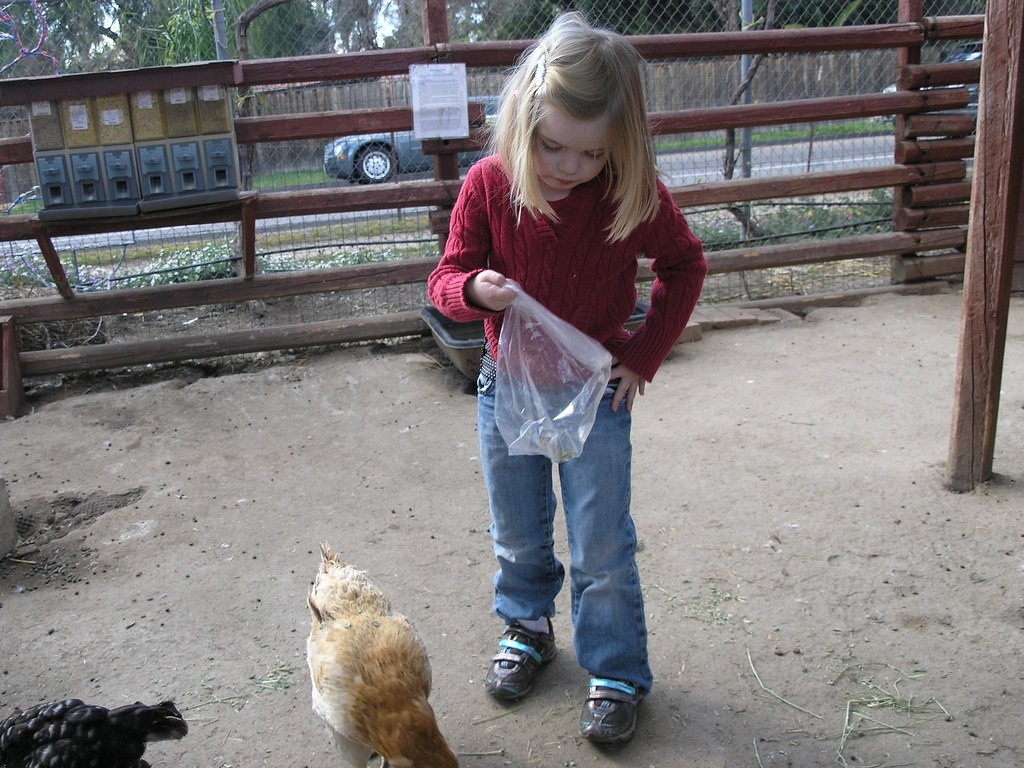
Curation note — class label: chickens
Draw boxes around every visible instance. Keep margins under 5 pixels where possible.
[304,541,462,768]
[0,697,187,768]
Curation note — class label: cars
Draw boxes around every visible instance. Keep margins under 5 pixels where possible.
[322,95,501,185]
[881,51,981,129]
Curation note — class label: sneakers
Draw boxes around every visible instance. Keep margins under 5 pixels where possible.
[483,618,557,699]
[578,677,638,743]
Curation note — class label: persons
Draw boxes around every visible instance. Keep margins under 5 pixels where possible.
[427,10,707,743]
